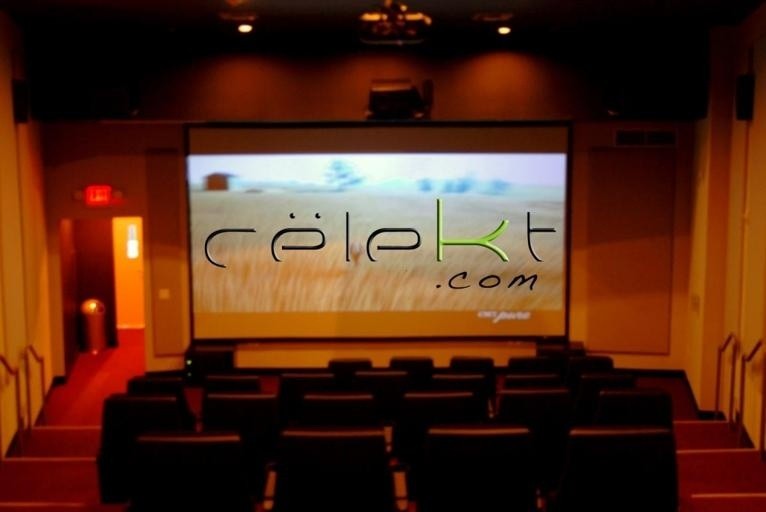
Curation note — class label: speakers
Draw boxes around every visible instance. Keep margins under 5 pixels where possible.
[735,73,755,121]
[12,78,30,122]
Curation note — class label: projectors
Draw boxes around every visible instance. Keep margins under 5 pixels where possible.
[358,12,432,45]
